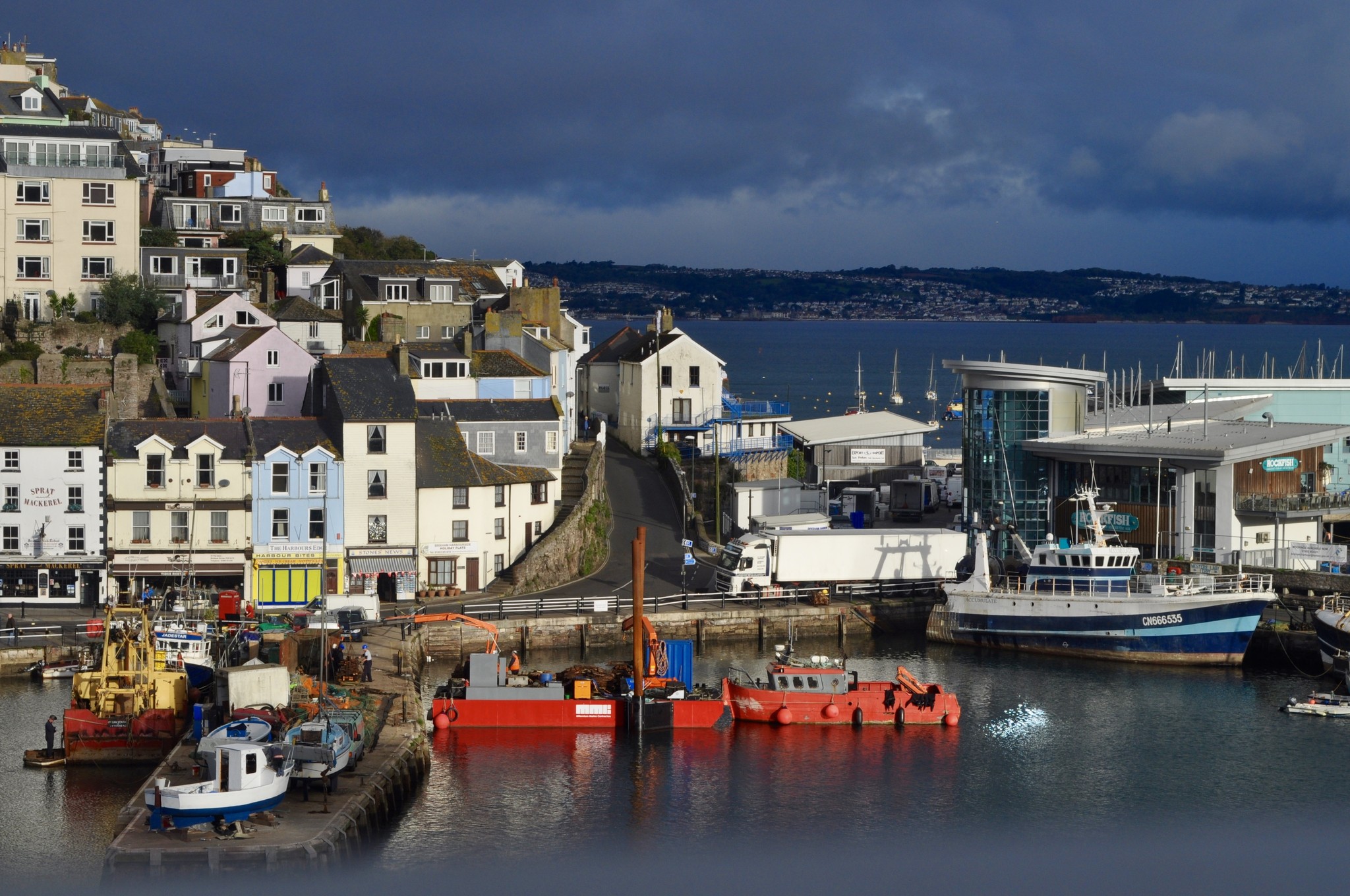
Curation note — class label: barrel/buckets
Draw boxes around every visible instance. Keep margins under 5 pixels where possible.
[565,695,569,699]
[192,765,200,776]
[850,511,864,529]
[155,777,166,789]
[540,673,552,683]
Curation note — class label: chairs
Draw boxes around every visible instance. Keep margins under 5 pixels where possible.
[82,159,89,167]
[1241,490,1350,512]
[186,219,195,230]
[64,158,72,167]
[99,160,106,167]
[22,157,32,165]
[204,218,213,230]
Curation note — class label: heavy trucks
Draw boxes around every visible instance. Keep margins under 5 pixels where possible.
[714,527,969,599]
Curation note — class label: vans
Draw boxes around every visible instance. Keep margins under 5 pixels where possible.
[336,606,370,643]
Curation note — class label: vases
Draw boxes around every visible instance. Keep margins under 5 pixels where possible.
[141,540,150,543]
[454,588,462,596]
[174,540,185,544]
[132,539,141,543]
[150,483,160,488]
[200,483,210,488]
[213,540,223,544]
[149,147,155,149]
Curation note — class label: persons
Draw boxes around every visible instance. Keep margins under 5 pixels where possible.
[244,601,254,619]
[332,644,345,680]
[164,588,178,611]
[267,746,285,776]
[45,715,57,759]
[947,492,954,512]
[209,583,239,594]
[507,650,520,675]
[354,645,373,682]
[742,578,754,606]
[141,588,154,610]
[462,655,470,686]
[5,613,16,646]
[581,416,591,443]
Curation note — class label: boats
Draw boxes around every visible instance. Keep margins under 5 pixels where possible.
[844,390,869,415]
[720,616,961,727]
[1282,592,1350,718]
[925,406,1279,667]
[27,491,375,833]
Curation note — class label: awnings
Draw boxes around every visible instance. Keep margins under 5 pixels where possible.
[348,558,417,577]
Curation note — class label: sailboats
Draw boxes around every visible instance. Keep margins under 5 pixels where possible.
[854,351,867,400]
[926,379,940,431]
[941,354,964,421]
[924,354,937,401]
[889,349,904,404]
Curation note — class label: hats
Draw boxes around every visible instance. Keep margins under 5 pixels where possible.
[50,715,57,720]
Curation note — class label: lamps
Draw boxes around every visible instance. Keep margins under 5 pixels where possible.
[53,581,61,596]
[566,391,575,398]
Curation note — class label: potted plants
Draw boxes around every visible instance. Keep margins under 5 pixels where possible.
[68,503,82,511]
[446,585,456,596]
[2,503,18,511]
[438,583,446,597]
[419,580,427,598]
[428,580,436,598]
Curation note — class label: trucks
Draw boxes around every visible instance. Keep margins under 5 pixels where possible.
[303,593,381,623]
[885,463,962,523]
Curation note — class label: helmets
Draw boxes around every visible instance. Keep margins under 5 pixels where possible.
[511,650,517,655]
[362,645,368,649]
[339,644,344,649]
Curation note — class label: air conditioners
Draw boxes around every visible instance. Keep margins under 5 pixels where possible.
[1258,531,1271,544]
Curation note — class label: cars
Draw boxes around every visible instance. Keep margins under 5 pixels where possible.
[925,460,939,467]
[944,462,963,477]
[282,610,317,632]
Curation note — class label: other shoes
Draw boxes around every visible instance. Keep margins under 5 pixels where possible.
[48,756,54,759]
[360,681,365,682]
